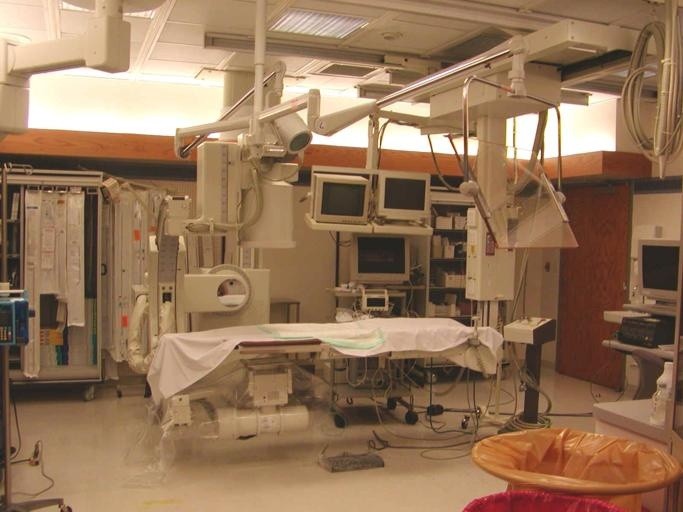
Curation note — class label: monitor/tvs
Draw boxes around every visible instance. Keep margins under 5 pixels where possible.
[637,239,680,307]
[378,170,430,219]
[313,173,370,225]
[352,233,410,284]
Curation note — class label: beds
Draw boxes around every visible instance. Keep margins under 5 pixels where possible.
[149,316,503,443]
